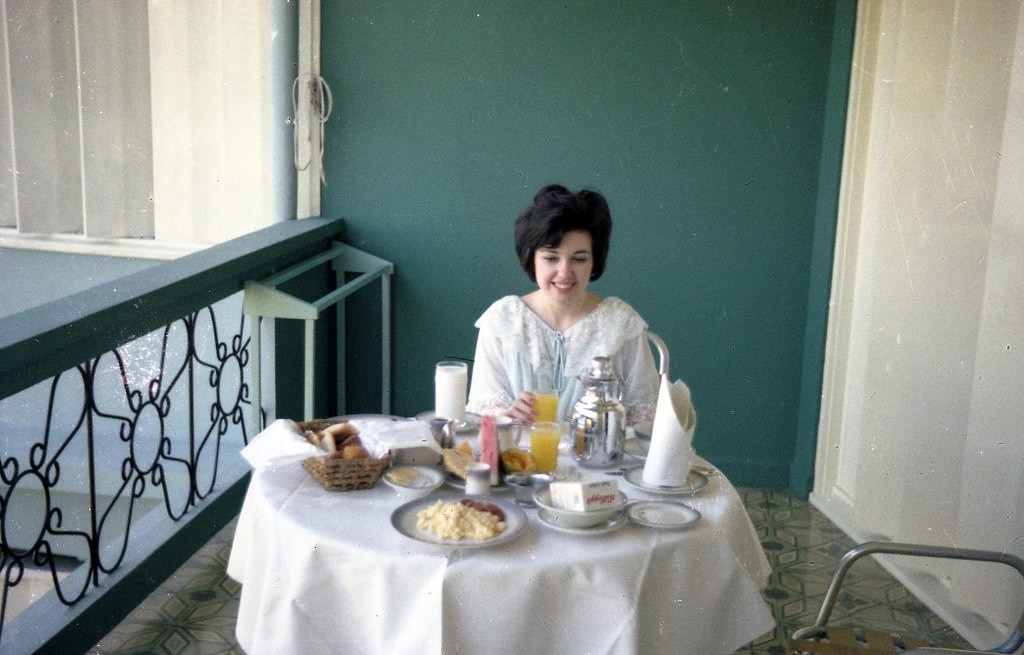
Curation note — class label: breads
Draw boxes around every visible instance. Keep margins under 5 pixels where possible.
[306,421,372,460]
[441,440,480,481]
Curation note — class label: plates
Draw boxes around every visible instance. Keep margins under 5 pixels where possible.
[445,474,509,491]
[623,464,708,494]
[623,500,701,528]
[391,495,528,547]
[536,507,628,534]
[416,411,483,432]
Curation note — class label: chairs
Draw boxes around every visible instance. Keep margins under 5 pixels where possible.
[784,542,1024,655]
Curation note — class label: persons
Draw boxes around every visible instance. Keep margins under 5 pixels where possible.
[465,184,660,425]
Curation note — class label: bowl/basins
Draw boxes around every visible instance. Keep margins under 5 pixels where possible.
[532,483,629,528]
[633,421,653,441]
[504,472,556,506]
[623,438,652,462]
[382,465,444,496]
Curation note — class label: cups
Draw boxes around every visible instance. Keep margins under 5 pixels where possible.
[530,422,561,473]
[436,361,467,427]
[533,388,558,423]
[465,462,491,495]
[428,419,465,449]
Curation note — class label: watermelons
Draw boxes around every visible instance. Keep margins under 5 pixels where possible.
[479,414,499,486]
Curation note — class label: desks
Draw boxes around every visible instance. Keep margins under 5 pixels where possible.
[224,411,778,655]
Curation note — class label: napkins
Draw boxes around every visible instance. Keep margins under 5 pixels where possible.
[642,375,696,487]
[239,418,327,471]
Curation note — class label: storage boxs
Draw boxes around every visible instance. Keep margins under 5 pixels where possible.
[550,478,620,513]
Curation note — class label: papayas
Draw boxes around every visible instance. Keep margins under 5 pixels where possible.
[500,448,537,474]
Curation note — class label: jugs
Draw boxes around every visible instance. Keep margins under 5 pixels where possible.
[568,356,627,468]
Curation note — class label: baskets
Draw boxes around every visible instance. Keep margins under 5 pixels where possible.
[295,419,390,491]
